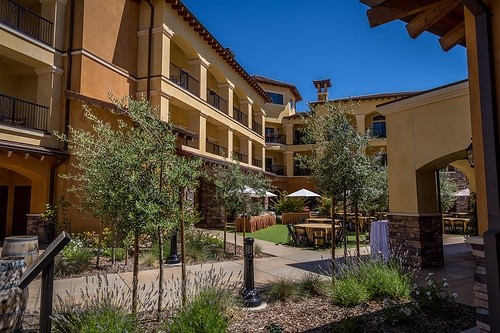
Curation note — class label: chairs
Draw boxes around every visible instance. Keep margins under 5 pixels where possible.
[312,228,328,251]
[327,228,344,248]
[287,223,310,247]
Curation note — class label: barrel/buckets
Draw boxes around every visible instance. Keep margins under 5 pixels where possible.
[0,256,29,333]
[1,236,39,271]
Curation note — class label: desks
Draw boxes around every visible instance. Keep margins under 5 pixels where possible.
[451,212,470,217]
[294,224,340,245]
[306,218,340,224]
[442,213,455,217]
[443,217,471,234]
